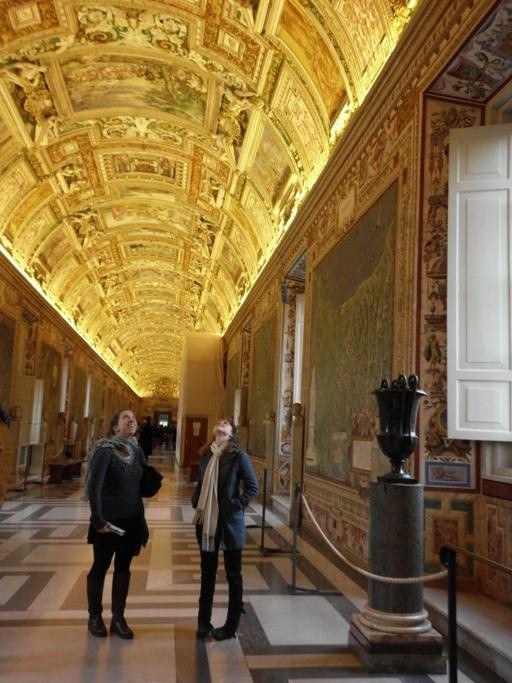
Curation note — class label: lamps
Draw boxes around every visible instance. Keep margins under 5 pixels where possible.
[0,405,22,428]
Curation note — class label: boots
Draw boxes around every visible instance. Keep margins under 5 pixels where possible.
[109,573,134,639]
[211,574,243,641]
[86,574,108,637]
[196,573,216,639]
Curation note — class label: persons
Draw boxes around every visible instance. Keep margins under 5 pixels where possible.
[133,416,176,461]
[86,409,148,637]
[192,416,260,640]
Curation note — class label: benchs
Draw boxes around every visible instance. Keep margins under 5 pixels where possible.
[47,459,81,484]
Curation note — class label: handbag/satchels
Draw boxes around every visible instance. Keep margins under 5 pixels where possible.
[140,459,163,497]
